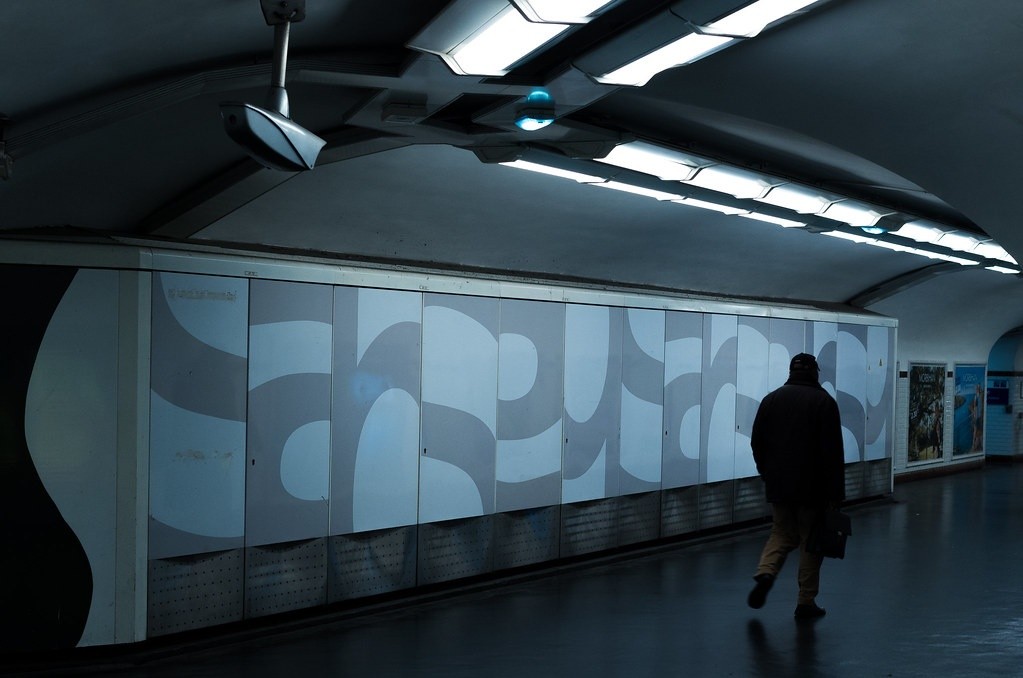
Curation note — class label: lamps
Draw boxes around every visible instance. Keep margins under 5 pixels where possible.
[452,133,1021,274]
[573,0,836,87]
[514,108,555,131]
[407,0,627,78]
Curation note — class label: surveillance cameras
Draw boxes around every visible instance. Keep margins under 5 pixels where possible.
[218,102,328,172]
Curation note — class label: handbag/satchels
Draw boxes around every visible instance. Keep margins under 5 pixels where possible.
[805,500,852,559]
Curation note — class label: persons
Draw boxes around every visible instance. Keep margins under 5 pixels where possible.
[746,353,846,618]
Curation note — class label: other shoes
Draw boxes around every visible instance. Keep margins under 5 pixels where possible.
[748,575,772,609]
[795,604,825,618]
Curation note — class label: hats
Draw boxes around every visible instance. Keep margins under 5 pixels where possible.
[790,353,820,371]
